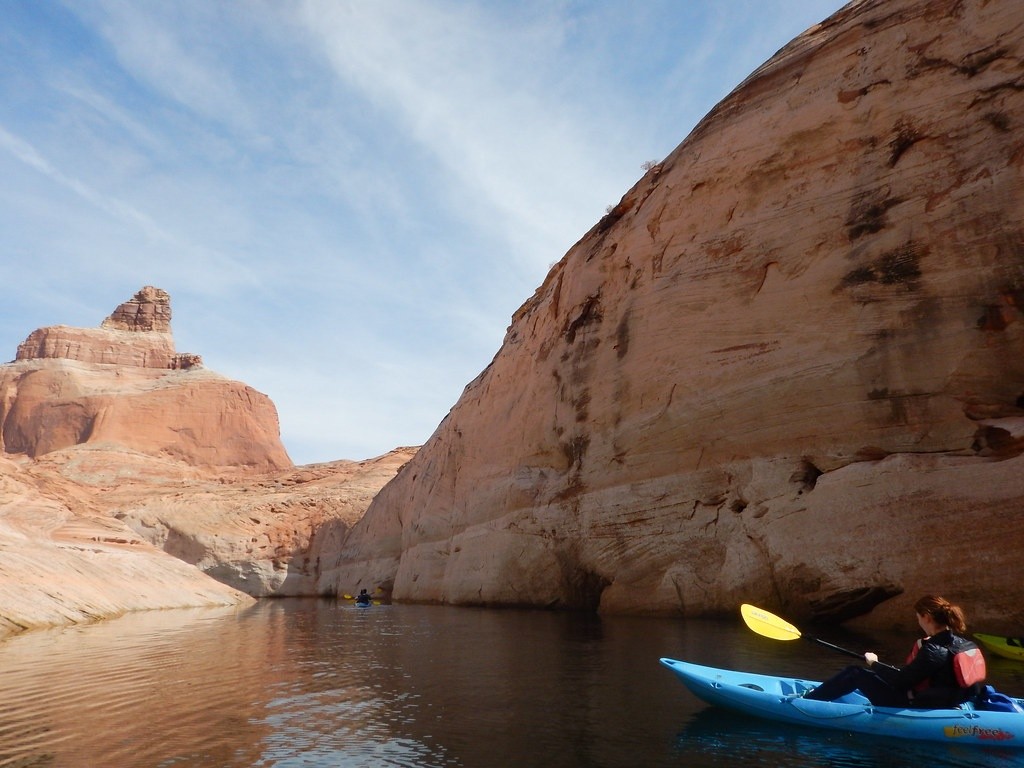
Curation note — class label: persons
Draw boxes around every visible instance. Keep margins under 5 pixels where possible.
[800,595,975,710]
[355,589,372,604]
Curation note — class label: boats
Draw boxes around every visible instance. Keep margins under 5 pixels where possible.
[660,657,1024,747]
[356,602,371,607]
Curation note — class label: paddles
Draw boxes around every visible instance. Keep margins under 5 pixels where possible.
[344,594,392,605]
[738,603,903,671]
